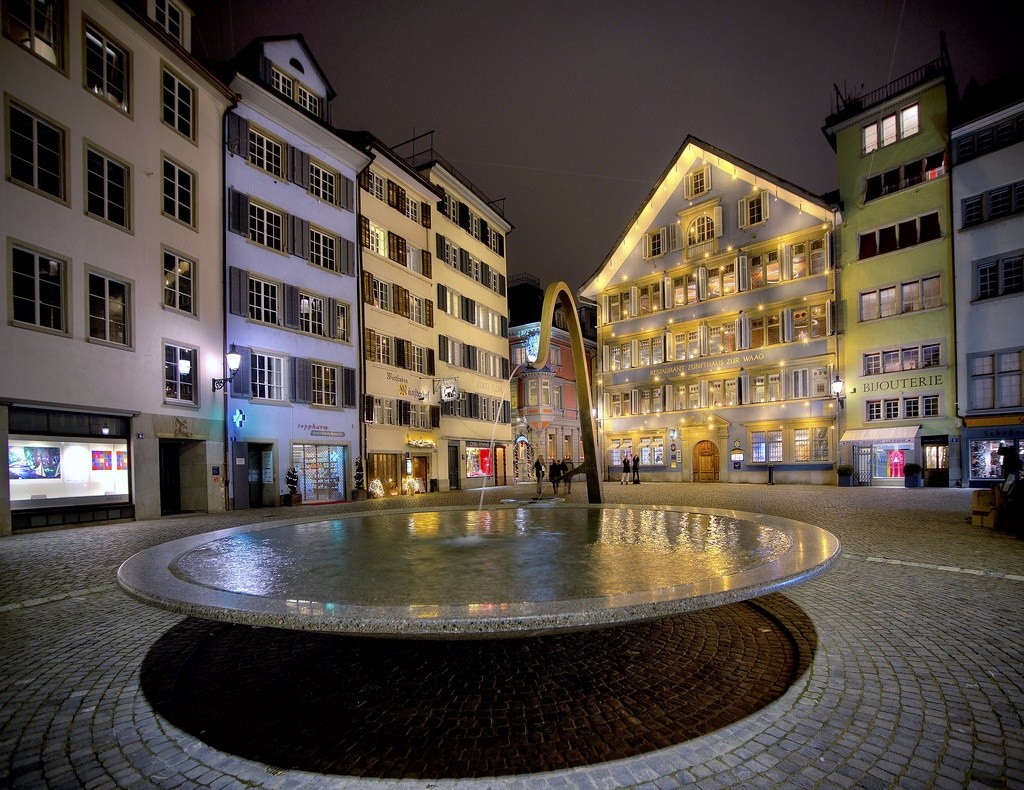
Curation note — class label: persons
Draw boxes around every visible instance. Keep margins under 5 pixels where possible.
[549,460,572,494]
[632,454,639,483]
[621,455,631,485]
[532,455,547,493]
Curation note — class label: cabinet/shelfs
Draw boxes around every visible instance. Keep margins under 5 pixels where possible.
[306,461,339,495]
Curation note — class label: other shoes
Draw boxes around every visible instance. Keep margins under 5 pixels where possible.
[621,482,623,485]
[554,491,556,494]
[626,482,628,485]
[568,491,571,494]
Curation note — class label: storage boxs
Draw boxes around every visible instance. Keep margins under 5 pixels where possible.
[972,490,996,506]
[968,505,998,530]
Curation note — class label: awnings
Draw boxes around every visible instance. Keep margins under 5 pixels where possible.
[840,425,921,445]
[607,442,663,450]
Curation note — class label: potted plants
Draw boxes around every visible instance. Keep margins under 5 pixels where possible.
[352,456,367,501]
[284,467,302,507]
[836,464,856,487]
[903,463,922,488]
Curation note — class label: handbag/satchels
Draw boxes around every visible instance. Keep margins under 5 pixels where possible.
[542,465,546,471]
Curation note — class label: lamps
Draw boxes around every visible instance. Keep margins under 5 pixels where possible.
[419,397,424,401]
[832,375,844,409]
[212,341,242,393]
[406,451,410,458]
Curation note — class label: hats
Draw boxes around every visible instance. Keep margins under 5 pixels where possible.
[552,460,556,463]
